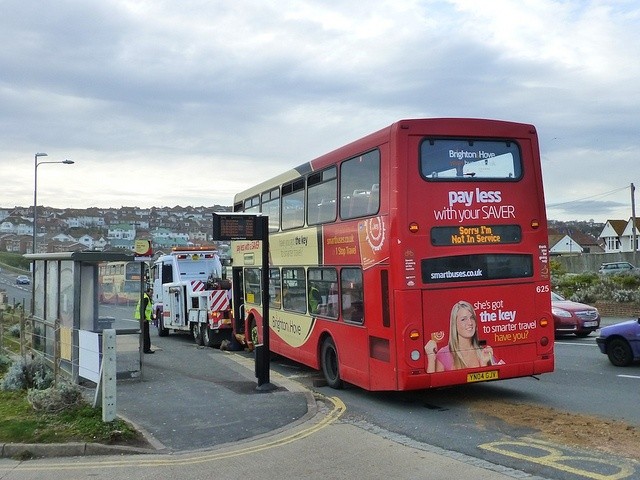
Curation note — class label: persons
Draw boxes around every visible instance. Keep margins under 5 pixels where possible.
[309,282,323,314]
[134,288,155,353]
[424,300,493,373]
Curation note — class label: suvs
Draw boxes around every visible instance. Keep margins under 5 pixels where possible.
[598,262,640,275]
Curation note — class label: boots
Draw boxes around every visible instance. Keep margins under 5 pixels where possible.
[220,340,228,350]
[225,339,231,344]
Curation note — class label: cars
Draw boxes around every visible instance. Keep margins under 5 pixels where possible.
[595,317,640,366]
[551,291,600,338]
[16,275,30,285]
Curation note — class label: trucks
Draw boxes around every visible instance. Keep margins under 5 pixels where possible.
[145,247,233,347]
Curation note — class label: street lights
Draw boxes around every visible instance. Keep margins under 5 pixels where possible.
[32,152,75,254]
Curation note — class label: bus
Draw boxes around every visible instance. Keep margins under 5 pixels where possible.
[98,261,150,306]
[231,118,554,391]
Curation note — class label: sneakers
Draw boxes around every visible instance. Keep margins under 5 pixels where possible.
[144,349,155,353]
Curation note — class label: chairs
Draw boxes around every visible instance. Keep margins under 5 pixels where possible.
[317,198,333,222]
[367,183,380,214]
[348,189,367,217]
